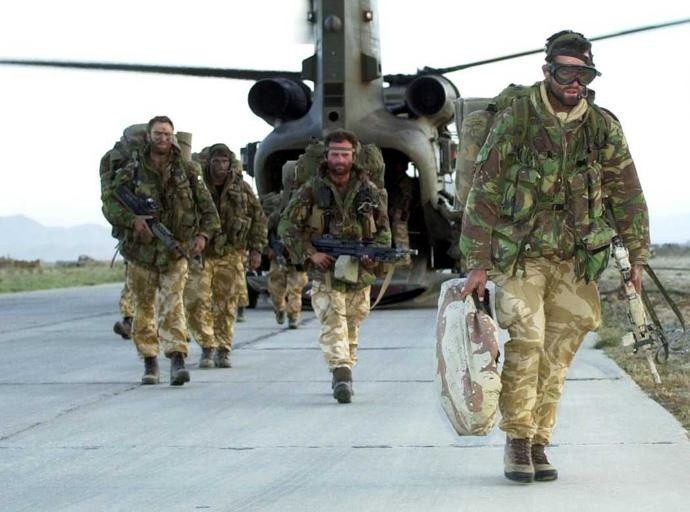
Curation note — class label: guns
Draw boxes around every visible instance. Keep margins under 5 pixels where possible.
[312,234,418,262]
[115,184,191,264]
[268,230,283,258]
[601,197,662,384]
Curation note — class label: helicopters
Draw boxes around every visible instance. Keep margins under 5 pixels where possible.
[0,0,690,316]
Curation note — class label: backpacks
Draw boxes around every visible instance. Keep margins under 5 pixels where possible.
[454,85,610,207]
[292,135,386,193]
[99,124,149,240]
[193,146,243,171]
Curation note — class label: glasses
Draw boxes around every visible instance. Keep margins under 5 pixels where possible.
[548,61,602,86]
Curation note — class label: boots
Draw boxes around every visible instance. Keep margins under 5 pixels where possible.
[114,317,133,339]
[503,433,536,482]
[532,443,557,482]
[142,355,160,385]
[331,367,354,403]
[276,309,298,328]
[169,350,190,386]
[199,346,215,368]
[214,345,233,367]
[237,306,247,322]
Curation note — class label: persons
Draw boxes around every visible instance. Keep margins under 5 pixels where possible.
[277,125,391,404]
[188,144,268,370]
[113,270,137,343]
[98,116,221,387]
[264,208,309,330]
[462,27,654,485]
